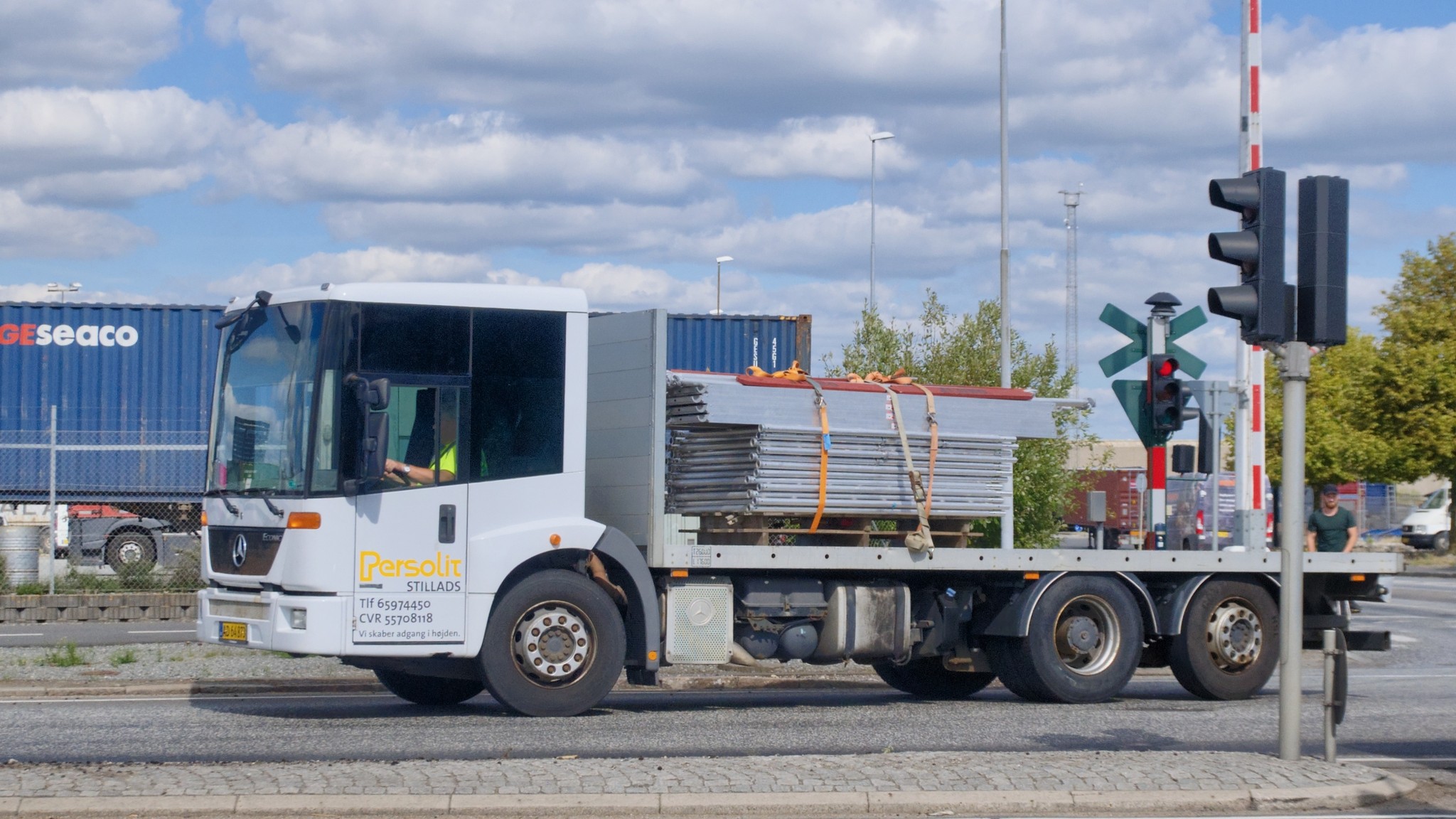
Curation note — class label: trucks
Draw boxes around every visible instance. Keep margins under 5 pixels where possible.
[0,300,323,581]
[1063,465,1147,550]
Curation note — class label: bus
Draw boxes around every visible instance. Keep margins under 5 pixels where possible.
[1164,467,1273,552]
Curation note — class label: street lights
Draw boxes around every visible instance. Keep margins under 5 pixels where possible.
[716,256,734,314]
[869,132,896,308]
[47,280,83,302]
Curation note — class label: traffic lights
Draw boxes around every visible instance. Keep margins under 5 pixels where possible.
[1208,166,1286,345]
[1150,351,1183,432]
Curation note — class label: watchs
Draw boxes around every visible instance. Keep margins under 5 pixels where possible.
[403,463,411,476]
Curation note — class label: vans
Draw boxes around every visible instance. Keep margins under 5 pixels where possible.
[1400,480,1453,554]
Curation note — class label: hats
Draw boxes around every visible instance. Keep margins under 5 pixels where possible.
[1322,484,1339,496]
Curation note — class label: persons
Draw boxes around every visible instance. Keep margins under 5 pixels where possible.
[380,404,488,488]
[768,520,786,546]
[1306,484,1359,631]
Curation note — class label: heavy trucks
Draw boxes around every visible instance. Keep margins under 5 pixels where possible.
[205,279,1406,719]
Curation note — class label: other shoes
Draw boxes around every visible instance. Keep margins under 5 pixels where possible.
[1349,602,1362,613]
